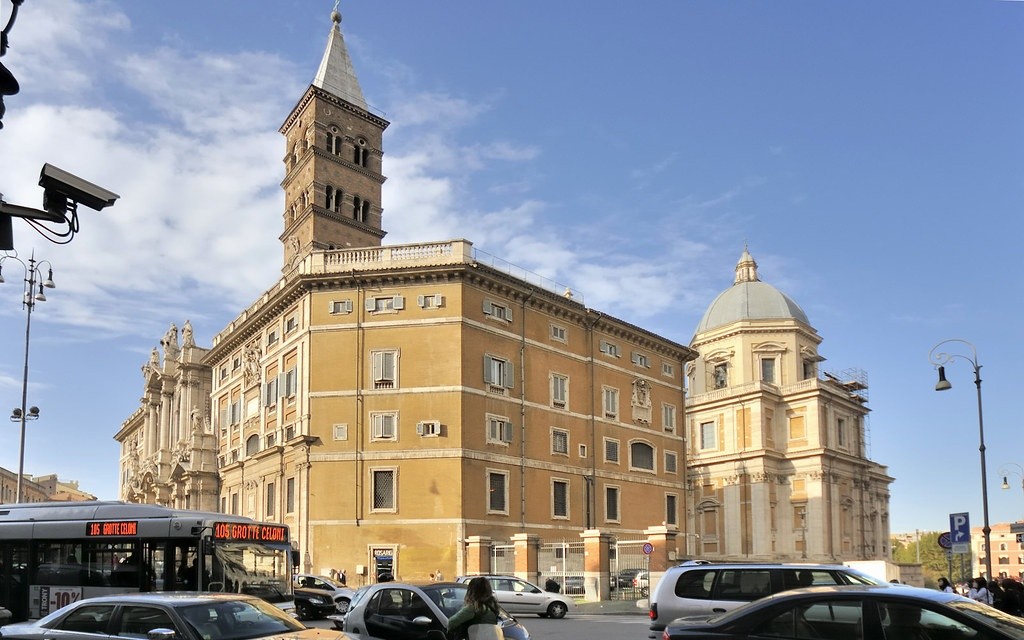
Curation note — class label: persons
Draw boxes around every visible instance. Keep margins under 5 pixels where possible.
[434,569,444,582]
[429,573,436,582]
[796,570,816,589]
[1,571,31,625]
[938,577,957,595]
[1,541,224,591]
[448,577,502,639]
[885,602,930,640]
[340,569,347,586]
[334,569,341,582]
[964,574,1024,618]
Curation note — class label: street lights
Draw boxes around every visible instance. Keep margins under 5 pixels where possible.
[930,338,992,583]
[0,246,56,504]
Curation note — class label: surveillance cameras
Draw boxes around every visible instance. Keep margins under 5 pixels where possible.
[39,164,120,213]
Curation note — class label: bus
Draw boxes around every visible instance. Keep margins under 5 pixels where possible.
[0,500,297,626]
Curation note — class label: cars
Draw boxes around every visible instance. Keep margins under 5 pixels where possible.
[0,593,381,640]
[294,558,1024,640]
